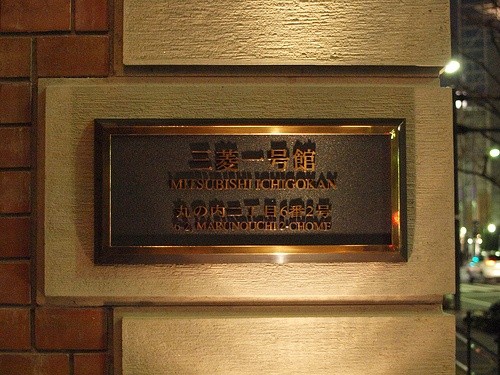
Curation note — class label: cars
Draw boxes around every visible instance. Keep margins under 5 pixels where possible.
[466,250,500,281]
